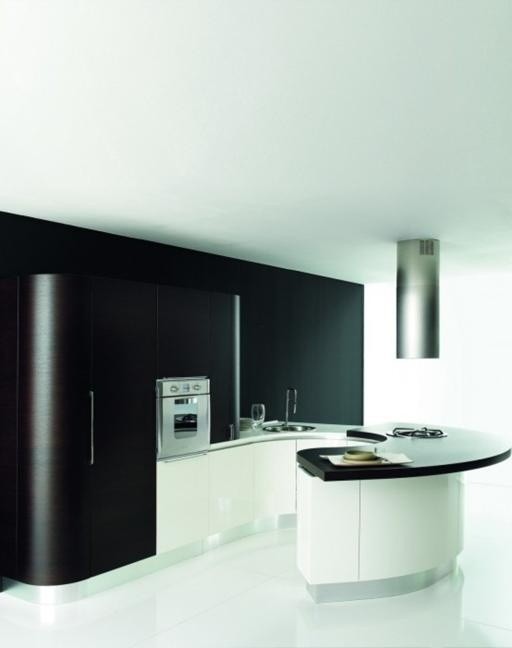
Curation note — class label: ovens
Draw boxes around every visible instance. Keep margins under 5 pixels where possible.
[156,376,210,459]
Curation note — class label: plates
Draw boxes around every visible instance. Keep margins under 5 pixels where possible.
[342,456,384,464]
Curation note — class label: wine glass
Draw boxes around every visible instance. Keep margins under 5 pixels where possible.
[251,403,265,434]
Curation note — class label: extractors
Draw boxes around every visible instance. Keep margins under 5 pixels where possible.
[397,239,440,359]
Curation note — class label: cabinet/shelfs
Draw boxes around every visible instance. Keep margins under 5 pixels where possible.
[0,273,241,586]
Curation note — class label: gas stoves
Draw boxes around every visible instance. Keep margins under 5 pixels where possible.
[386,426,448,438]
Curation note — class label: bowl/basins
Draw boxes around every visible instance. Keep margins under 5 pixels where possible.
[345,450,373,460]
[240,418,254,431]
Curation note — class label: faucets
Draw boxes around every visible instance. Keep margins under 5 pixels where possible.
[284,386,297,422]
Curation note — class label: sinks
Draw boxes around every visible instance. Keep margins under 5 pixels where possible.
[262,425,316,431]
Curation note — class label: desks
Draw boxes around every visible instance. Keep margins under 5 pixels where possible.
[295,423,511,604]
